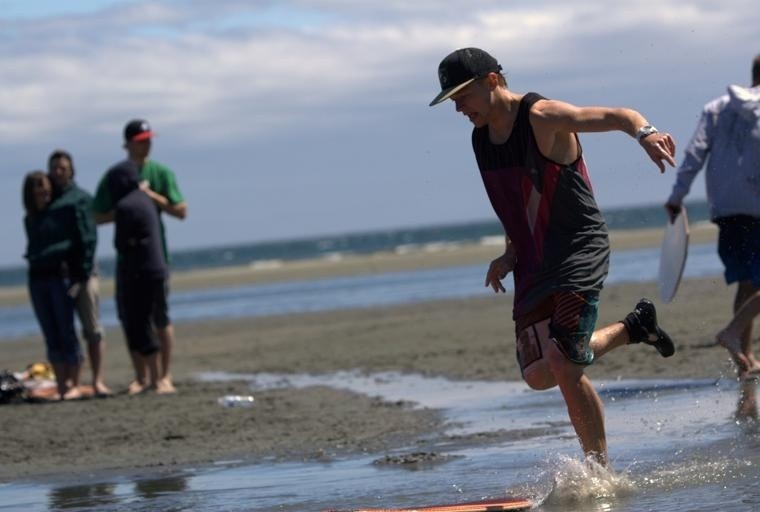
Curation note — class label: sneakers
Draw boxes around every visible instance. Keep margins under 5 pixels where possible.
[634,299,675,357]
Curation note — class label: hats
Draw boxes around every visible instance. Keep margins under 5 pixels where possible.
[429,47,502,106]
[126,119,157,142]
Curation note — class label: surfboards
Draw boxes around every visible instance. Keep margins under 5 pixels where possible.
[362,499,533,511]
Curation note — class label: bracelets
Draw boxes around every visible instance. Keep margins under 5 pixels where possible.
[636,125,658,143]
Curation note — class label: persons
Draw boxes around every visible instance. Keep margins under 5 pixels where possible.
[107,161,176,394]
[49,152,114,398]
[428,47,677,477]
[93,118,186,394]
[666,55,760,380]
[23,169,85,400]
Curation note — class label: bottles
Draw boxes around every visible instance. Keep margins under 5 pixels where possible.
[216,395,256,408]
